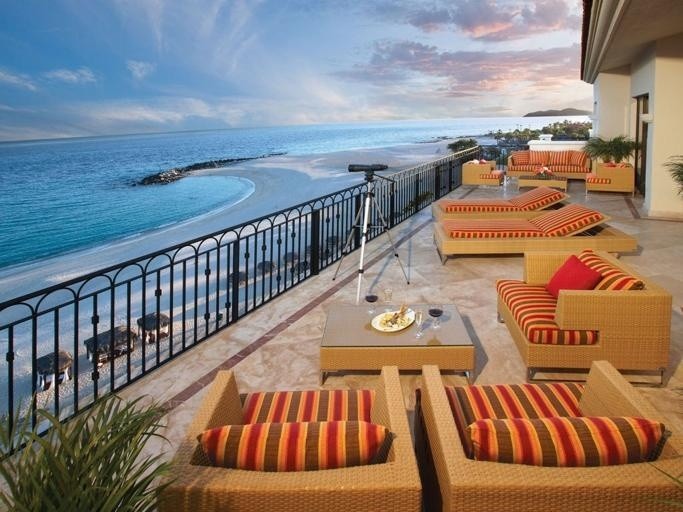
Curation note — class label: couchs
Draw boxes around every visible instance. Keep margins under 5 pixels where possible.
[506,150,591,183]
[493,248,674,388]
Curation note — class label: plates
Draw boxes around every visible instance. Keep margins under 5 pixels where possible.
[370,308,415,333]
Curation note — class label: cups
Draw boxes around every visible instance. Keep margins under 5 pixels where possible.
[383,289,392,303]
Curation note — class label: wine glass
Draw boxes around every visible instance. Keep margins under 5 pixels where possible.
[364,288,378,313]
[413,309,424,339]
[428,301,443,329]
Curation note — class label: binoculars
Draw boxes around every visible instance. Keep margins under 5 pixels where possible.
[349,164,395,183]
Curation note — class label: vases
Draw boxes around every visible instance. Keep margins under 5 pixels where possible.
[537,174,551,179]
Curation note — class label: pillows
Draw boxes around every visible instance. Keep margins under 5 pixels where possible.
[546,255,605,298]
[469,159,486,164]
[512,151,585,166]
[605,162,625,169]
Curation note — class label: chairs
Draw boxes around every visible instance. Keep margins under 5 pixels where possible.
[431,186,611,266]
[462,159,505,186]
[416,360,683,512]
[160,364,422,510]
[586,161,635,197]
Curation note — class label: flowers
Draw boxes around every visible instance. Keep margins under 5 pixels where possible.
[534,166,554,175]
